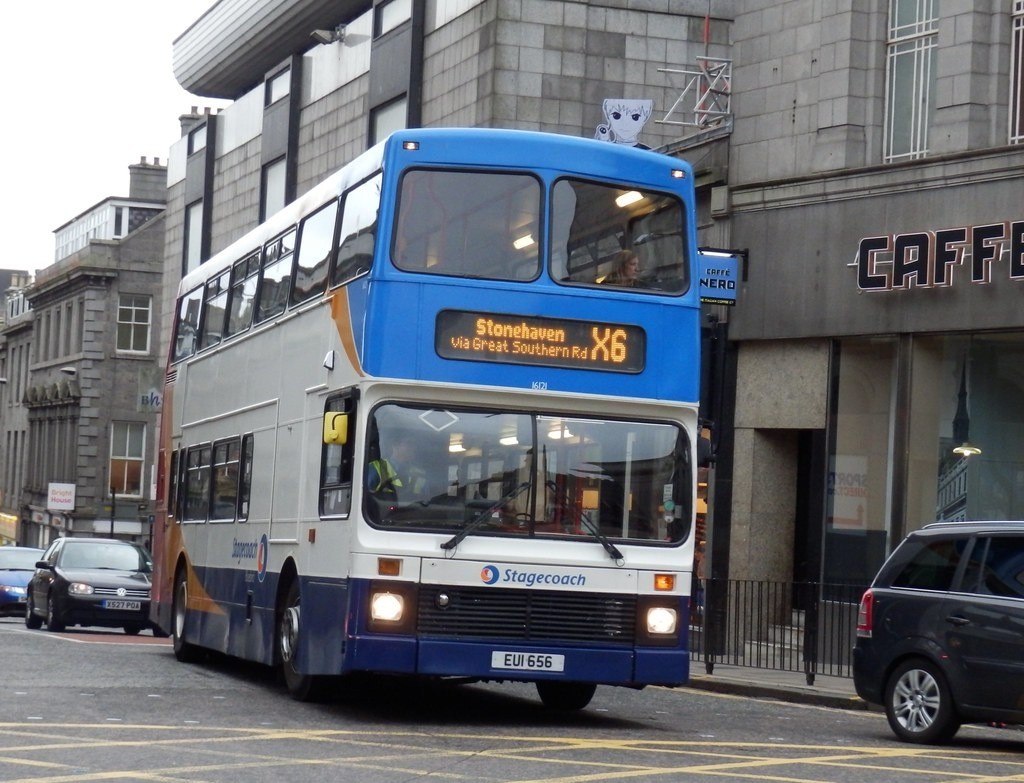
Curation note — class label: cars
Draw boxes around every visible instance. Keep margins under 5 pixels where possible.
[0,546,47,619]
[24,537,169,638]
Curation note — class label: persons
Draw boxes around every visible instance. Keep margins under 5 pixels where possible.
[367,433,434,498]
[601,250,659,290]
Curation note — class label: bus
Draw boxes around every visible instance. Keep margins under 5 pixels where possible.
[150,124,724,715]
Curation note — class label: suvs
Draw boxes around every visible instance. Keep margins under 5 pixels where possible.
[851,520,1024,746]
